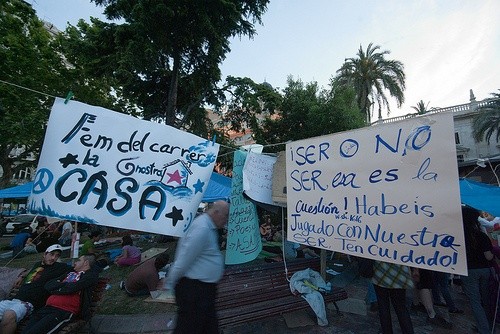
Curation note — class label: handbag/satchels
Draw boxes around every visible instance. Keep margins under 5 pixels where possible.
[358,257,375,278]
[366,279,377,304]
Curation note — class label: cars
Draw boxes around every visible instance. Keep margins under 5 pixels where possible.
[6,215,44,234]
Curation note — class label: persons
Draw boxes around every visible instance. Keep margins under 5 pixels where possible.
[18,253,99,334]
[167,200,229,334]
[0,244,73,334]
[360,259,419,334]
[416,267,464,328]
[113,236,141,266]
[79,231,110,267]
[259,216,317,262]
[11,217,73,256]
[458,207,500,334]
[21,207,26,214]
[119,255,166,296]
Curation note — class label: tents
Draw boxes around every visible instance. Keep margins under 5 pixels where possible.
[202,172,232,203]
[0,181,33,217]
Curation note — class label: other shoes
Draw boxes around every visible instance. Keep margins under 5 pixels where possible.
[410,302,425,312]
[448,308,465,315]
[452,278,461,286]
[427,313,452,330]
[119,281,125,290]
[433,302,448,309]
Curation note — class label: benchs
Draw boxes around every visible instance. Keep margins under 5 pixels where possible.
[216,256,349,334]
[7,273,111,334]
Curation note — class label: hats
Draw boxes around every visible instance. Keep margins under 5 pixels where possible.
[45,244,63,256]
